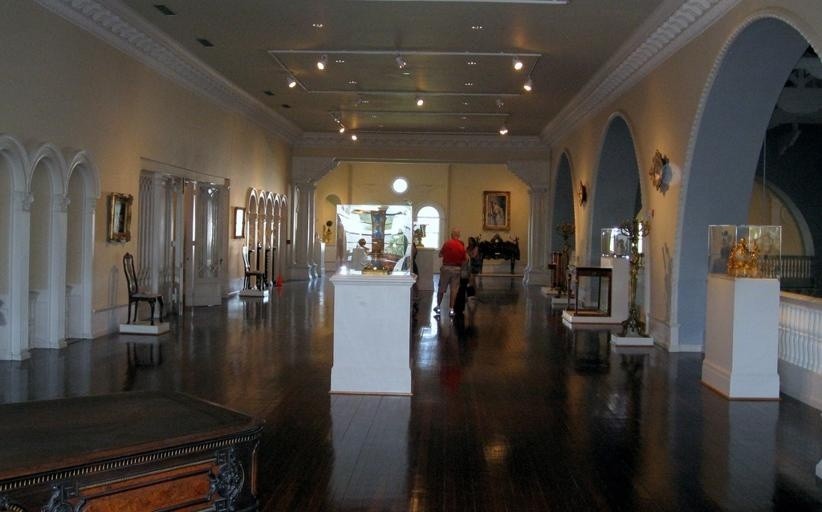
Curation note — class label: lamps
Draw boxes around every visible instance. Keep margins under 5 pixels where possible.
[280,50,536,141]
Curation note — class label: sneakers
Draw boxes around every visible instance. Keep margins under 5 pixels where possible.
[433,306,441,316]
[449,308,457,316]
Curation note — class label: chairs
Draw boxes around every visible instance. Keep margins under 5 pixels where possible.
[121,250,166,325]
[242,244,266,291]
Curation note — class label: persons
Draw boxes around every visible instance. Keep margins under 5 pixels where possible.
[412,242,421,301]
[355,239,373,269]
[433,227,481,317]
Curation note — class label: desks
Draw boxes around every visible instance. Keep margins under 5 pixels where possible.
[567,264,613,317]
[2,386,267,511]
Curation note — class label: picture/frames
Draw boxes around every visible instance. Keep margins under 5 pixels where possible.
[107,190,134,243]
[482,189,511,232]
[234,206,246,238]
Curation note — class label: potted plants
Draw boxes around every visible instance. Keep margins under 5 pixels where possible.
[556,223,576,296]
